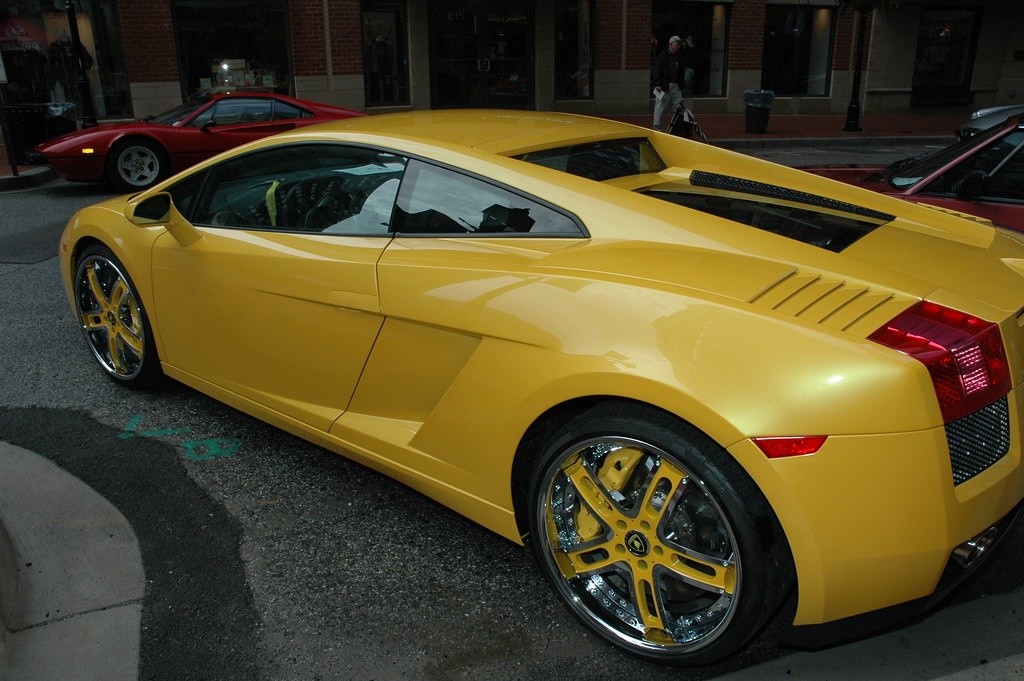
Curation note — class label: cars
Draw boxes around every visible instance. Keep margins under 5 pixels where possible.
[953,99,1024,144]
[777,110,1024,239]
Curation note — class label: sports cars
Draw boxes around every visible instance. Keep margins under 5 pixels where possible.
[23,90,380,192]
[59,105,1024,673]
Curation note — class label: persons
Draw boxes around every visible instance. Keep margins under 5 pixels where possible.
[48,35,94,130]
[651,36,694,130]
[681,35,696,68]
[782,28,809,95]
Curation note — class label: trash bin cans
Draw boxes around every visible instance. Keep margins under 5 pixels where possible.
[744,90,775,134]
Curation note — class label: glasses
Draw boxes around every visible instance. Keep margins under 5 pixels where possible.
[676,40,681,43]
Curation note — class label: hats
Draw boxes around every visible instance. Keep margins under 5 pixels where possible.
[669,36,682,43]
